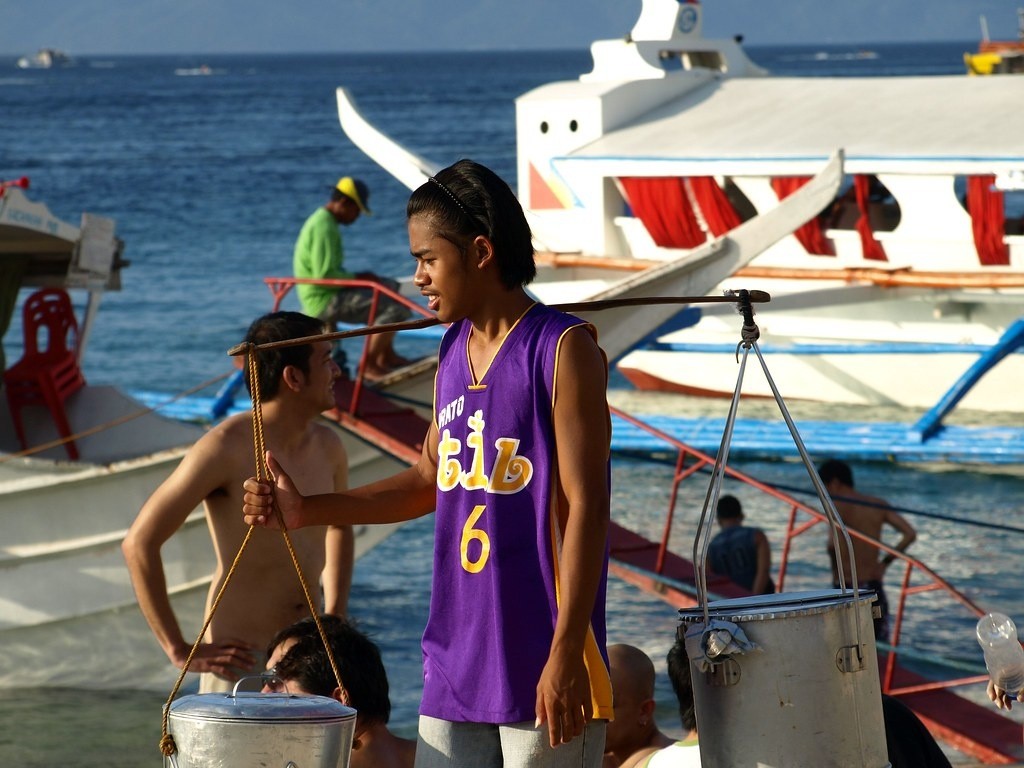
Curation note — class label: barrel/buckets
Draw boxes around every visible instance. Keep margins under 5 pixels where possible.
[162,675,358,768]
[678,588,893,768]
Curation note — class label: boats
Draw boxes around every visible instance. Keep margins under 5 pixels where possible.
[2,144,844,695]
[325,0,1024,416]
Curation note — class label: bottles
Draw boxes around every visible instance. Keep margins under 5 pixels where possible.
[976,611,1024,700]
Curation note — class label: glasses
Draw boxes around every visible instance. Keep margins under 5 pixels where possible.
[260,668,281,689]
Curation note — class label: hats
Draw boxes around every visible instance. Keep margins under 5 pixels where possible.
[335,176,371,217]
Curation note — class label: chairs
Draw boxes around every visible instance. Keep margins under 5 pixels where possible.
[0,287,86,461]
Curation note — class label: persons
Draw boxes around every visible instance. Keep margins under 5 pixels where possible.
[260,614,417,768]
[121,311,355,695]
[292,176,416,381]
[705,495,775,595]
[243,159,613,768]
[602,642,702,768]
[816,458,917,643]
[987,665,1024,710]
